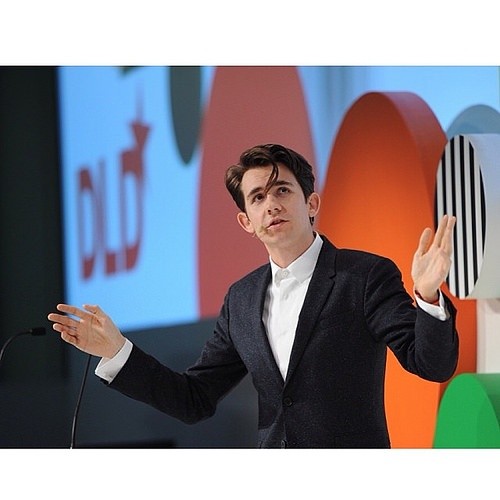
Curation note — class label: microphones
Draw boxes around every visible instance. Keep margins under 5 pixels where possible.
[251,225,263,238]
[0,326,46,367]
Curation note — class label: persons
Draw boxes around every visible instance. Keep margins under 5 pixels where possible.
[46,144,460,449]
